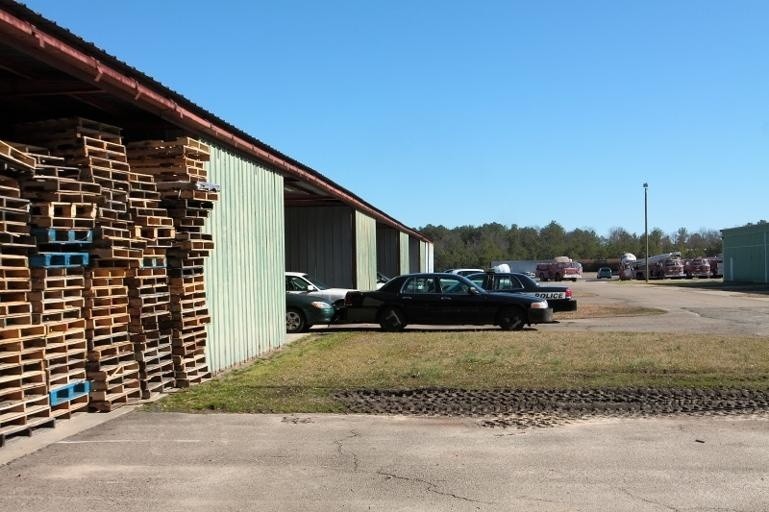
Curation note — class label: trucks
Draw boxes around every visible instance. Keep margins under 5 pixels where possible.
[534,261,583,282]
[621,251,724,280]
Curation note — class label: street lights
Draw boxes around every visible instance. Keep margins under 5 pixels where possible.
[642,181,649,284]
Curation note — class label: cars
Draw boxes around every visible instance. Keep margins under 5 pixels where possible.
[597,267,612,279]
[285,268,577,331]
